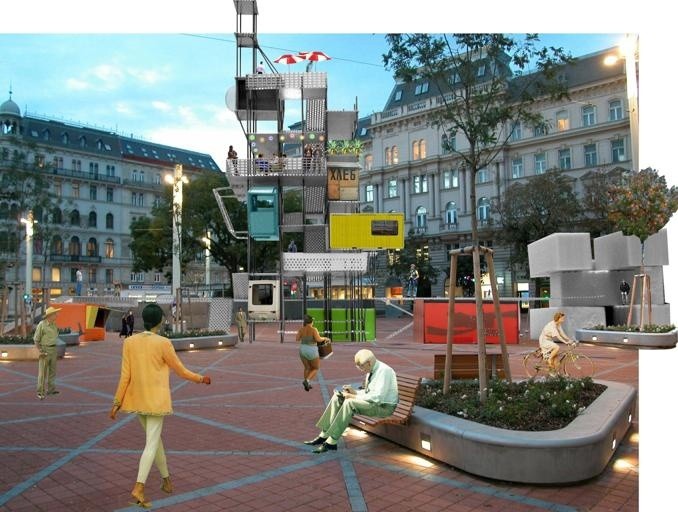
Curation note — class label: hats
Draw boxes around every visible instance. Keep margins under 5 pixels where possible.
[42,307,63,318]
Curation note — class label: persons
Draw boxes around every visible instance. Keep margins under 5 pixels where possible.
[303,348,399,455]
[305,59,314,71]
[254,152,272,176]
[226,145,238,176]
[271,152,279,163]
[109,302,211,509]
[117,312,127,339]
[311,144,323,174]
[32,306,63,401]
[619,280,630,306]
[73,266,84,297]
[255,60,265,74]
[111,279,122,297]
[295,314,330,393]
[406,263,420,297]
[301,143,312,175]
[287,239,298,252]
[537,312,573,376]
[233,307,248,343]
[278,153,287,170]
[126,311,134,338]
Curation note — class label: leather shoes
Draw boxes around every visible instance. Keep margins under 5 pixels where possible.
[304,437,337,453]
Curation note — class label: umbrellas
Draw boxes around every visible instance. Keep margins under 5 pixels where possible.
[299,50,333,71]
[272,52,305,70]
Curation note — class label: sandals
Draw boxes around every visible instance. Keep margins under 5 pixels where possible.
[128,500,151,508]
[160,486,172,493]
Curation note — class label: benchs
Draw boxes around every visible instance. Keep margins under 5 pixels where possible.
[434,355,509,381]
[352,374,422,427]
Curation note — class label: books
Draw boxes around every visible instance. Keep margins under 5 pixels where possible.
[332,385,358,399]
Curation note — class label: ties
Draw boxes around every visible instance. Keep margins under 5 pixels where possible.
[365,373,372,393]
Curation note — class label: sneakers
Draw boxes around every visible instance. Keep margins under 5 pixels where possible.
[302,379,312,391]
[37,391,61,399]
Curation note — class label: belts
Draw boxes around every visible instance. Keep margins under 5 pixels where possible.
[48,345,55,347]
